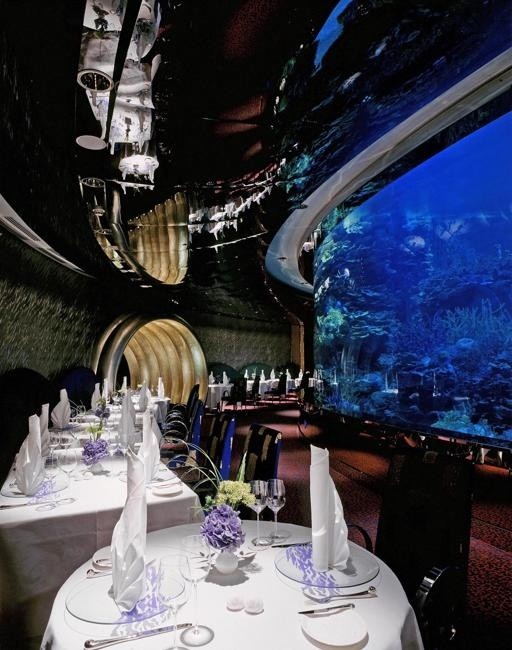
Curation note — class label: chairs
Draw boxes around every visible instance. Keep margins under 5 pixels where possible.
[347,445,475,649]
[208,371,296,416]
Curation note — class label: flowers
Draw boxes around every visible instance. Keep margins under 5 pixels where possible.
[166,439,257,549]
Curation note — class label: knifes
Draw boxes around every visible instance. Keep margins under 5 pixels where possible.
[84,623,192,650]
[298,603,354,615]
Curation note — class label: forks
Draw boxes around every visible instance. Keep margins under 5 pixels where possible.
[303,586,376,603]
[86,559,156,579]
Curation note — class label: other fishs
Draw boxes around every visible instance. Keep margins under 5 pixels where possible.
[344,278,357,293]
[342,267,351,277]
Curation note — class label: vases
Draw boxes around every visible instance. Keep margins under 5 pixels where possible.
[215,549,238,574]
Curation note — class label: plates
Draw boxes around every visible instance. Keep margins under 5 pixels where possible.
[92,545,112,569]
[301,603,368,647]
[63,572,180,625]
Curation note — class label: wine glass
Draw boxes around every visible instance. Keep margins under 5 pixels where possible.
[264,478,286,544]
[157,554,193,650]
[2,385,146,506]
[175,534,215,647]
[247,480,269,551]
